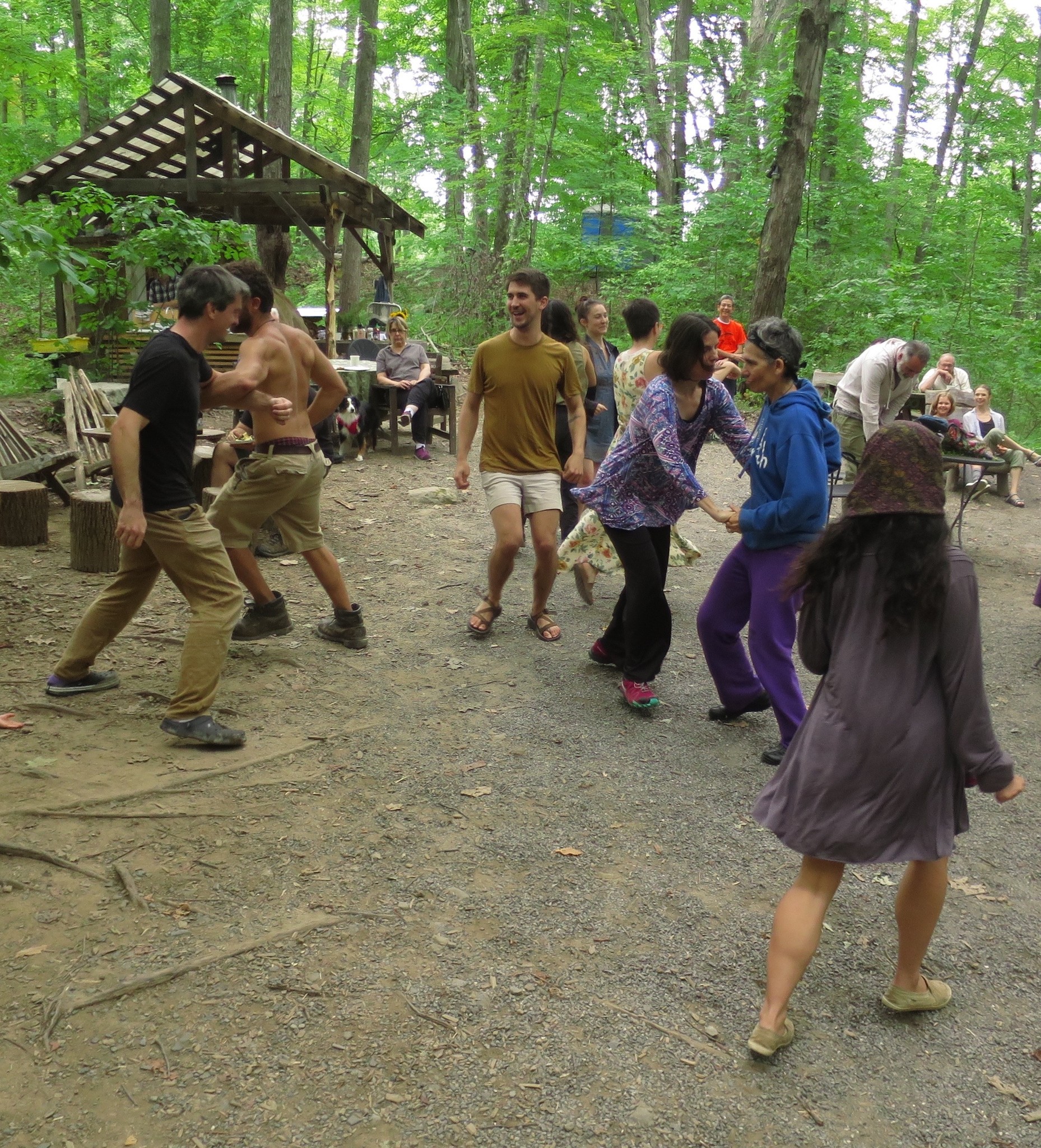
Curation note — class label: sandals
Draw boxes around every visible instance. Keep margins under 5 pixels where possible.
[527,608,563,641]
[1026,451,1041,466]
[468,595,502,635]
[1005,493,1025,506]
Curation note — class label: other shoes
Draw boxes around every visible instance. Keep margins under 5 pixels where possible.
[709,693,771,720]
[761,740,787,765]
[748,1018,794,1056]
[160,716,246,746]
[333,454,343,463]
[882,975,952,1012]
[971,484,991,500]
[965,479,988,491]
[45,671,120,696]
[574,562,596,605]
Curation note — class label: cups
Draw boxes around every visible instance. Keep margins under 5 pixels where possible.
[350,355,360,366]
[101,414,118,432]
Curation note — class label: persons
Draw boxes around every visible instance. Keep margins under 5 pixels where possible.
[746,419,1022,1055]
[48,259,1041,764]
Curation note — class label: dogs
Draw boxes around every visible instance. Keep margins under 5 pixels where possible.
[336,396,377,462]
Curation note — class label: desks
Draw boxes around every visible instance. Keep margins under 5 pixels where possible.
[315,338,430,358]
[940,446,1005,551]
[309,360,378,445]
[428,357,459,432]
[82,426,229,483]
[813,369,846,406]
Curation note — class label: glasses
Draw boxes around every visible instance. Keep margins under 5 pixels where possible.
[388,329,406,334]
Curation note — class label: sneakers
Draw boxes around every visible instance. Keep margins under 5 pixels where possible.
[256,533,292,557]
[312,603,368,648]
[618,676,658,708]
[398,411,412,427]
[414,445,432,461]
[231,590,295,641]
[589,637,614,664]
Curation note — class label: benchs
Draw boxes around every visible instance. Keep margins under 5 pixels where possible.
[49,381,131,429]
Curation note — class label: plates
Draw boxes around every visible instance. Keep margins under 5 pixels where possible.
[344,366,370,371]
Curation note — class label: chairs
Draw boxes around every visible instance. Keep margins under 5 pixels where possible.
[51,365,121,493]
[0,409,80,505]
[346,339,457,455]
[827,456,863,525]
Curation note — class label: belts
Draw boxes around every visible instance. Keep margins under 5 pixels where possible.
[256,443,320,455]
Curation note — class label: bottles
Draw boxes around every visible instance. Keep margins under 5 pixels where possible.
[196,410,203,435]
[352,323,387,341]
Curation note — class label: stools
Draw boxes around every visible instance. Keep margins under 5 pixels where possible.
[0,480,50,545]
[200,484,224,513]
[190,444,217,485]
[69,488,125,572]
